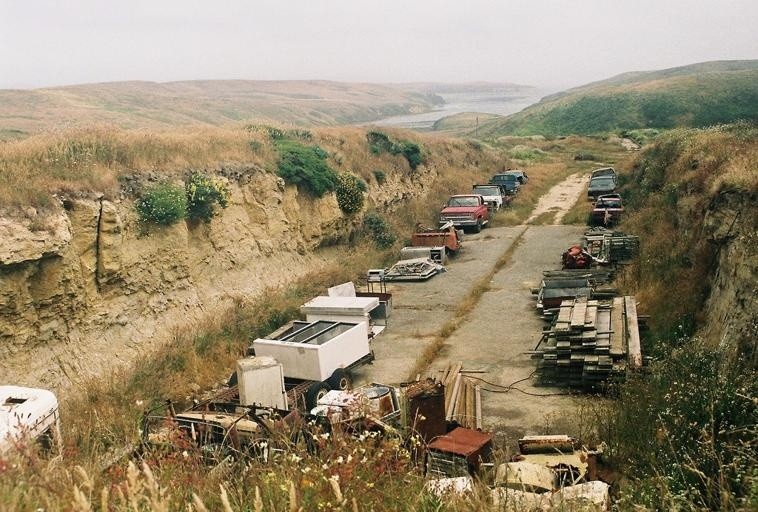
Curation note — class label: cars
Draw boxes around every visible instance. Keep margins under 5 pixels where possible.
[588,166,624,223]
[440,174,520,233]
